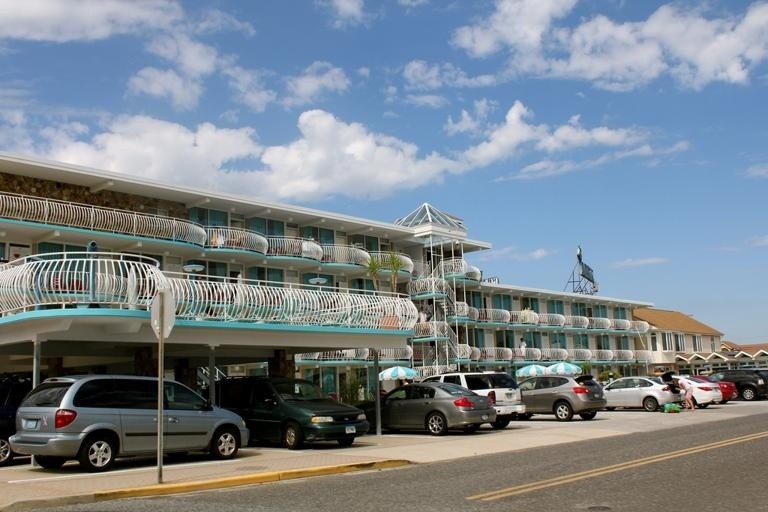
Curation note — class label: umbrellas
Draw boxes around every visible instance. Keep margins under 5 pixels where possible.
[516,365,552,379]
[379,365,419,380]
[547,362,583,376]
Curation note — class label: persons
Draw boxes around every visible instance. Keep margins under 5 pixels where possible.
[653,367,664,377]
[402,379,409,386]
[518,337,529,359]
[307,235,314,240]
[675,378,695,412]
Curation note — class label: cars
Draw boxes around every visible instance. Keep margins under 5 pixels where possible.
[201,376,370,450]
[355,370,607,435]
[600,370,767,411]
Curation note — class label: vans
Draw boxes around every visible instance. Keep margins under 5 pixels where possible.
[9,374,250,471]
